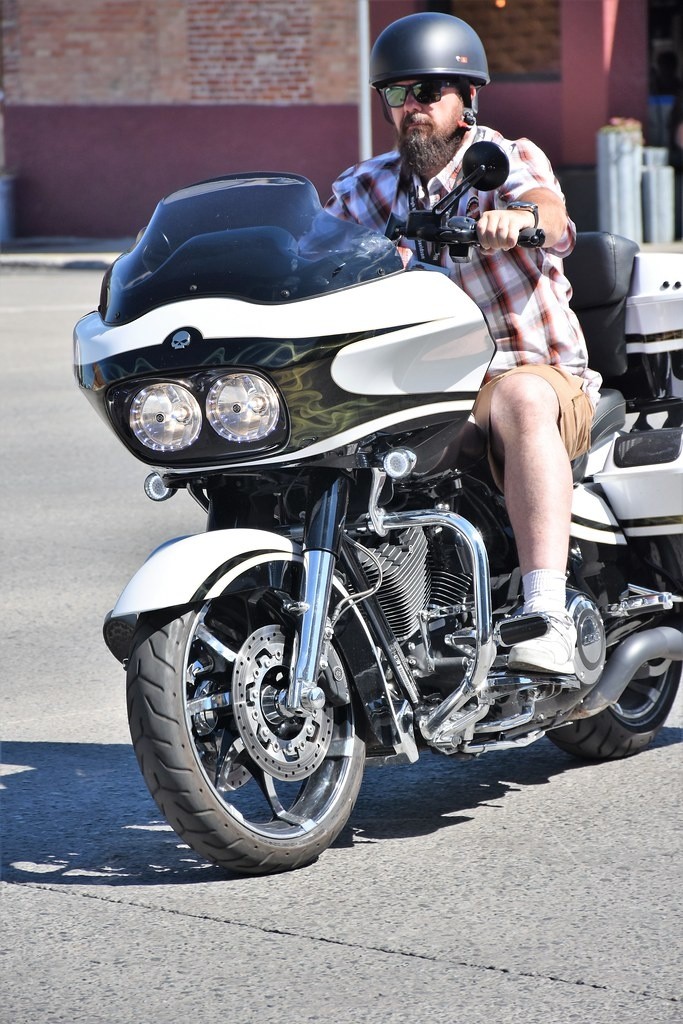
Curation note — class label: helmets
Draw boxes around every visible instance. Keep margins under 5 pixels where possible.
[369,12,491,88]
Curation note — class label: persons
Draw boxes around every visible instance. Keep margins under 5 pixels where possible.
[292,11,601,673]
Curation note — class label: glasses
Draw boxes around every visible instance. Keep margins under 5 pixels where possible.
[379,80,457,107]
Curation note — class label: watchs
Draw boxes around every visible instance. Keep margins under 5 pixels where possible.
[505,201,542,228]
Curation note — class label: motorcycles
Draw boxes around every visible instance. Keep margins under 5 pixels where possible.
[71,139,683,876]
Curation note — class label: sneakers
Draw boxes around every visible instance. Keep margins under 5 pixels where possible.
[508,596,578,674]
